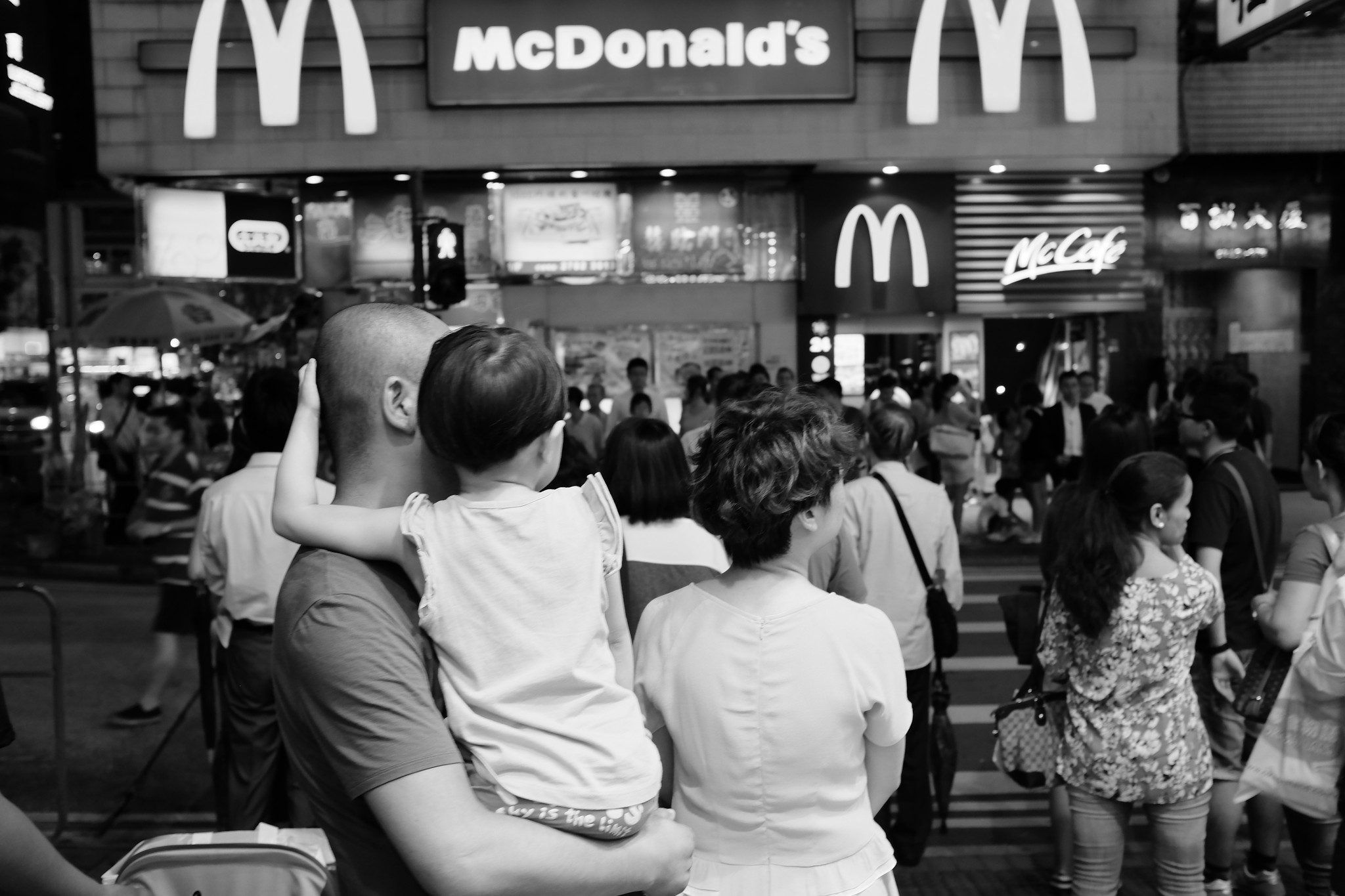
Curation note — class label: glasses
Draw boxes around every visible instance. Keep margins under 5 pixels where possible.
[1174,409,1201,421]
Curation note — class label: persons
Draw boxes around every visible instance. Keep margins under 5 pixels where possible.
[0,299,1345,896]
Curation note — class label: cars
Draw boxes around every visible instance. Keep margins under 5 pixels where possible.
[0,375,63,460]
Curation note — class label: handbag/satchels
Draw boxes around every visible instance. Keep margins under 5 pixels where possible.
[1288,525,1345,704]
[927,585,958,658]
[999,586,1044,663]
[929,423,975,456]
[98,442,115,469]
[1232,640,1292,722]
[993,664,1065,787]
[101,821,339,896]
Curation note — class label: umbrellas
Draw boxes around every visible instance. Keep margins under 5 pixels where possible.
[52,284,264,407]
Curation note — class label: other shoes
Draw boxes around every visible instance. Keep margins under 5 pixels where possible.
[111,703,163,725]
[1048,874,1072,889]
[1231,862,1278,885]
[988,533,1004,542]
[1203,868,1231,890]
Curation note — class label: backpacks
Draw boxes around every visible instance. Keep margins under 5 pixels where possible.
[1020,409,1050,482]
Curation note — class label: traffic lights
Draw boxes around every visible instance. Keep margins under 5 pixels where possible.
[424,220,469,311]
